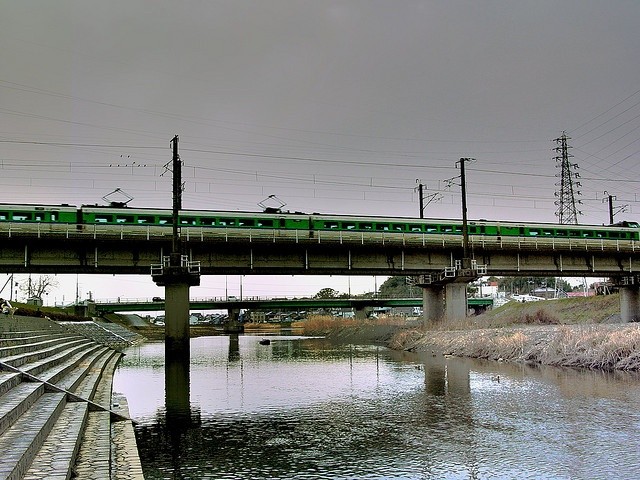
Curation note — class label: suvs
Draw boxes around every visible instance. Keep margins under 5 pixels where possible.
[153,297,165,302]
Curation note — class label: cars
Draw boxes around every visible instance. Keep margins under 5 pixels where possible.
[228,296,237,301]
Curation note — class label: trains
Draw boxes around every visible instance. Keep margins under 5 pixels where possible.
[0,204,640,241]
